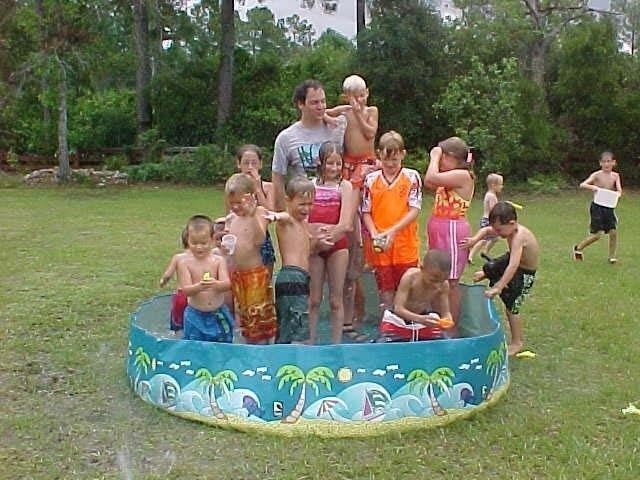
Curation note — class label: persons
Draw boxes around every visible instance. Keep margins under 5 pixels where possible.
[573,151,622,265]
[272,78,370,343]
[458,201,538,356]
[309,139,353,345]
[211,218,236,316]
[363,130,423,321]
[424,136,475,337]
[260,175,331,346]
[161,227,192,337]
[378,248,453,343]
[320,75,378,233]
[220,173,277,346]
[182,215,233,344]
[468,173,503,264]
[236,143,274,277]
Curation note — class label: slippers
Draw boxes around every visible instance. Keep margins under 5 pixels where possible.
[342,323,366,342]
[609,258,617,263]
[573,245,584,261]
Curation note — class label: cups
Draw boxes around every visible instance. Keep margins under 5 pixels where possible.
[223,235,236,254]
